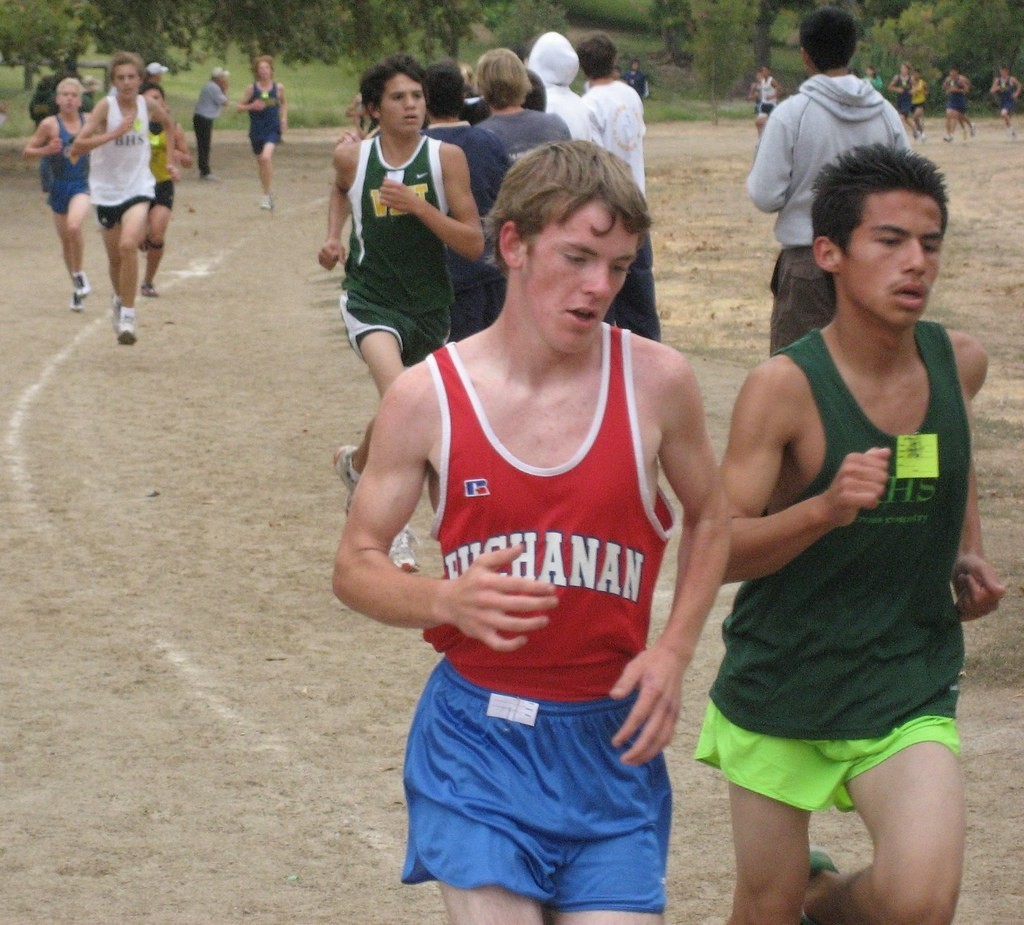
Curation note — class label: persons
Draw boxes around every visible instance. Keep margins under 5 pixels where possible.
[238,54,288,211]
[138,84,193,298]
[0,98,9,128]
[335,141,730,925]
[747,8,915,357]
[31,55,168,131]
[716,143,1006,925]
[749,60,1021,143]
[23,78,91,311]
[317,32,651,576]
[73,51,181,347]
[194,67,230,180]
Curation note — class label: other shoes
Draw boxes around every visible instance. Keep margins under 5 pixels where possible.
[912,131,923,141]
[944,134,954,142]
[200,174,218,179]
[962,127,974,142]
[141,284,159,296]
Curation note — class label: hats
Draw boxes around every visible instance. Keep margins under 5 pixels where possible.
[146,62,168,74]
[210,66,230,80]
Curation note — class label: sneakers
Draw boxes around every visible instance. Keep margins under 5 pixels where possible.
[116,315,137,345]
[261,196,272,209]
[73,271,89,297]
[71,289,81,311]
[333,447,361,516]
[388,523,420,573]
[801,848,840,925]
